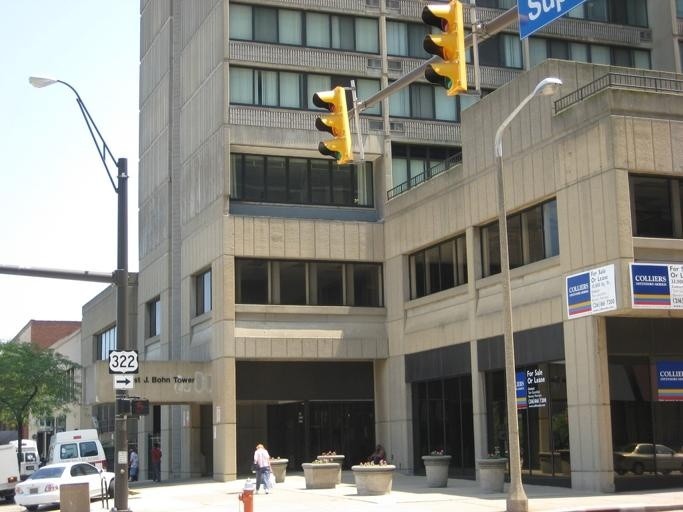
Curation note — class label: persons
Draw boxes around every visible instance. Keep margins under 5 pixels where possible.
[127,448,137,480]
[254,444,272,494]
[150,443,161,481]
[368,444,384,462]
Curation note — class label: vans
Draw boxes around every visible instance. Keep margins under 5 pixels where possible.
[46,429,107,472]
[7,439,42,476]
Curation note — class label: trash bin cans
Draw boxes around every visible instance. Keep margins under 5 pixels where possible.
[60,482,90,511]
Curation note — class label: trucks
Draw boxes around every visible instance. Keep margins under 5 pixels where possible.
[0,443,21,500]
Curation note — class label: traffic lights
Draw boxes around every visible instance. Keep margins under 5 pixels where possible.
[311,87,355,166]
[421,1,467,99]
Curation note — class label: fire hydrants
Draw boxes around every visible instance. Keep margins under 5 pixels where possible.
[239,478,254,511]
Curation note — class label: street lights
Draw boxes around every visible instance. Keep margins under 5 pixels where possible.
[26,75,130,510]
[494,77,564,510]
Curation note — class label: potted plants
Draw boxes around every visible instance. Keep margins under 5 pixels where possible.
[271,450,508,496]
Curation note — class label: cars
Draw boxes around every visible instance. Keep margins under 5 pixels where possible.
[612,442,683,474]
[13,462,115,511]
